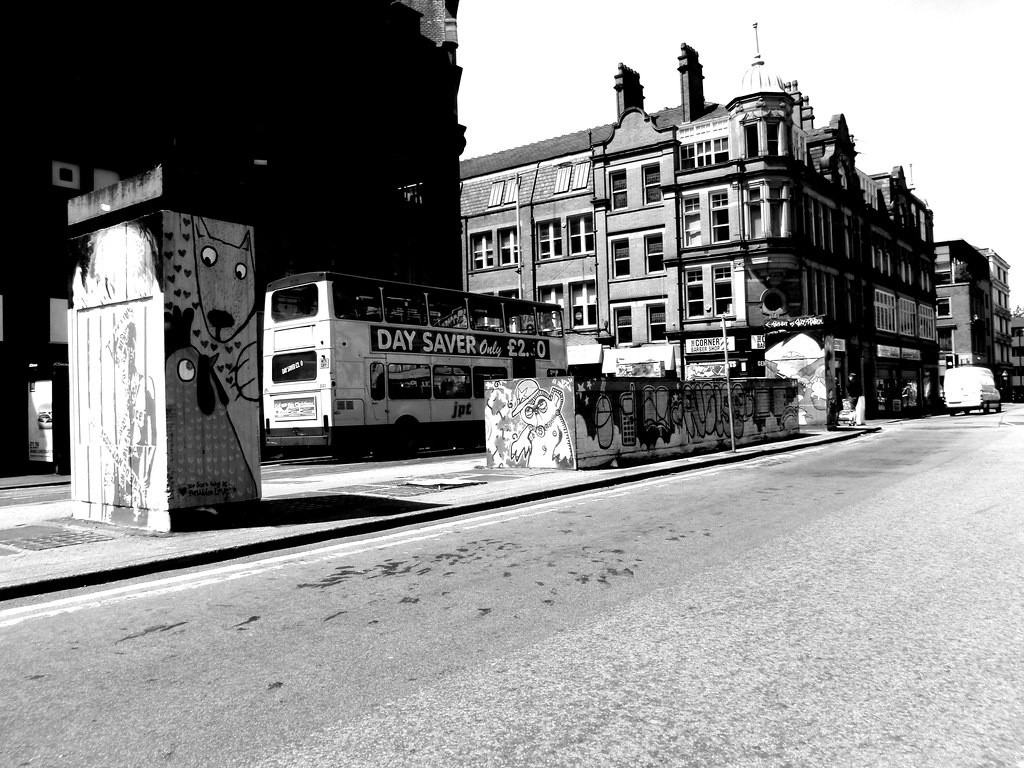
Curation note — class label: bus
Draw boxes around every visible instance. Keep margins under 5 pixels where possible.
[262,272,567,462]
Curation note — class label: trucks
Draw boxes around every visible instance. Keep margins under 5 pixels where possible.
[942,366,1003,416]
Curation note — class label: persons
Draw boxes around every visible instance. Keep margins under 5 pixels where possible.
[546,318,554,328]
[372,364,385,400]
[509,317,516,333]
[441,373,458,396]
